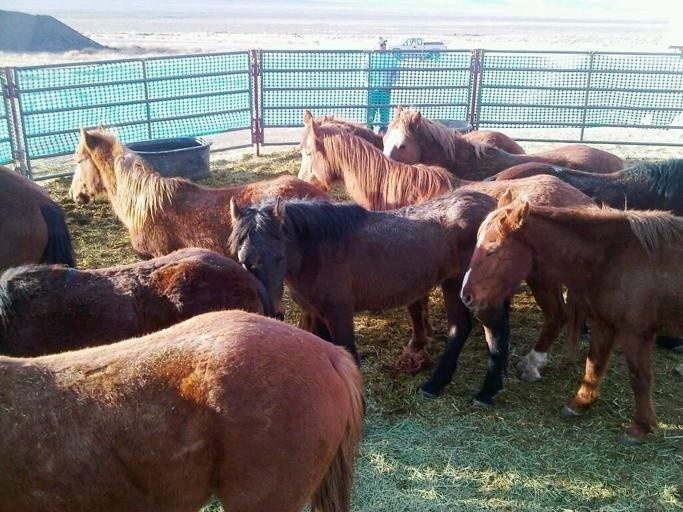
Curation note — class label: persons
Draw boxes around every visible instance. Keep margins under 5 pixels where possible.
[361,35,400,131]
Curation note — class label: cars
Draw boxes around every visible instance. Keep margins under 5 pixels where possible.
[391,37,448,60]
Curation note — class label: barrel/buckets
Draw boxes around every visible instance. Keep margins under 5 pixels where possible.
[432,117,469,134]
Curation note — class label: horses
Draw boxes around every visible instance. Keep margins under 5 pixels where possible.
[0,307,368,512]
[461,125,527,158]
[0,245,274,364]
[295,109,482,368]
[230,188,511,409]
[382,106,625,178]
[478,160,682,382]
[0,164,75,276]
[461,185,682,446]
[65,119,332,261]
